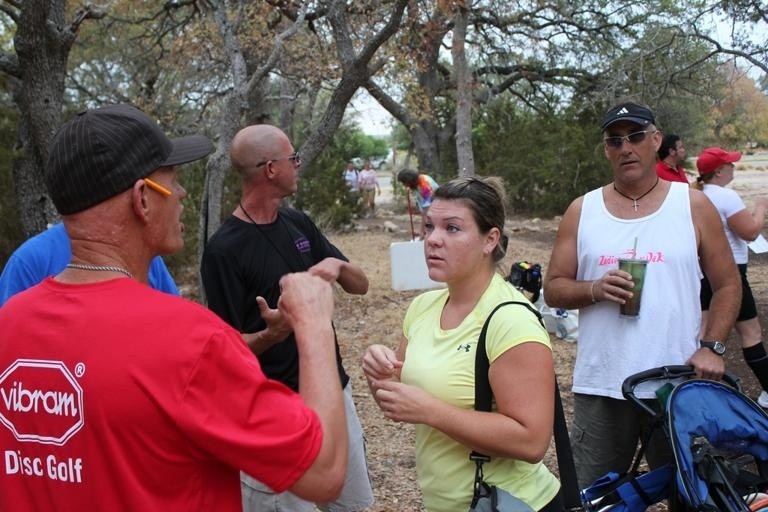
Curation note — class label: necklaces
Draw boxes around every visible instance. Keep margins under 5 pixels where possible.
[240,199,307,272]
[613,177,659,212]
[67,263,134,280]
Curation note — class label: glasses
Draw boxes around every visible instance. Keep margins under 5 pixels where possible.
[257,151,300,170]
[605,130,659,147]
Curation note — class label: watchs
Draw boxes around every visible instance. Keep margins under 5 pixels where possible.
[699,341,726,355]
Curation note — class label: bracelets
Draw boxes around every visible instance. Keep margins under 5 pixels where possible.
[590,279,599,304]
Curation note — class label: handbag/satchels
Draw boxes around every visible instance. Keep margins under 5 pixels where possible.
[469,301,582,511]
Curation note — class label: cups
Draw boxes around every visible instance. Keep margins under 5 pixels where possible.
[618,258,649,317]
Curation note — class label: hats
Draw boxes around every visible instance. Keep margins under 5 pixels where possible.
[43,103,213,214]
[697,147,742,176]
[601,101,656,134]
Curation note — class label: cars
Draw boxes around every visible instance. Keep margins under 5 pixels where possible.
[351,158,390,170]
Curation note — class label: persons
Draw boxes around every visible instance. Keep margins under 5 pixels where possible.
[1,223,183,305]
[398,168,439,240]
[362,176,562,511]
[696,148,768,409]
[200,123,374,511]
[0,103,351,511]
[657,134,703,191]
[342,162,359,209]
[358,162,381,219]
[543,101,742,492]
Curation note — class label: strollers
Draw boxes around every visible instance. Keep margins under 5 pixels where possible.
[587,363,768,511]
